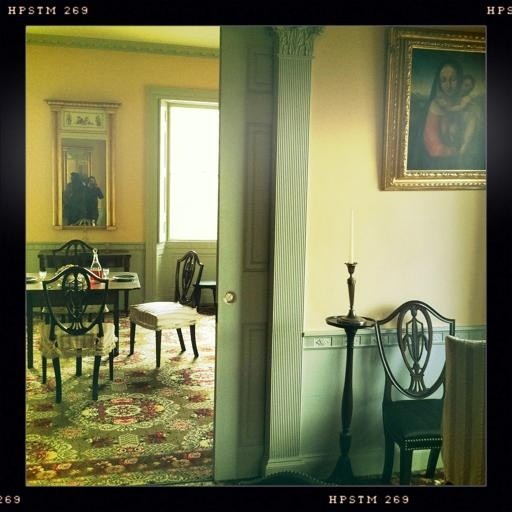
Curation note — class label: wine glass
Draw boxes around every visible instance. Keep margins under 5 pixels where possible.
[103,268,109,280]
[38,271,46,285]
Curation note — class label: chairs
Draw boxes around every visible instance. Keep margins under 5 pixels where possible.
[131,250,203,367]
[369,298,460,484]
[39,239,117,403]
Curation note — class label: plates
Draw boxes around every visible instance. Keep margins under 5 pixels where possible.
[113,275,136,281]
[26,276,37,284]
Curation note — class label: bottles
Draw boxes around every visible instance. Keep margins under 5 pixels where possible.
[89,248,101,284]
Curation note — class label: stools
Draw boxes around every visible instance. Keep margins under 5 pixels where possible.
[193,280,218,313]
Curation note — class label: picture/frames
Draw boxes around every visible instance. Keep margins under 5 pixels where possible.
[377,26,493,191]
[62,144,95,193]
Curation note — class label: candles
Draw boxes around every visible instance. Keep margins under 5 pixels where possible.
[348,209,356,265]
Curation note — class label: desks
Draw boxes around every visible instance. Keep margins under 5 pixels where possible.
[37,247,133,319]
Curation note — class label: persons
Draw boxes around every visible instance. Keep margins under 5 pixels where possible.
[424,59,484,169]
[62,172,104,226]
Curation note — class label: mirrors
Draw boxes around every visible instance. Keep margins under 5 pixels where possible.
[45,97,123,232]
